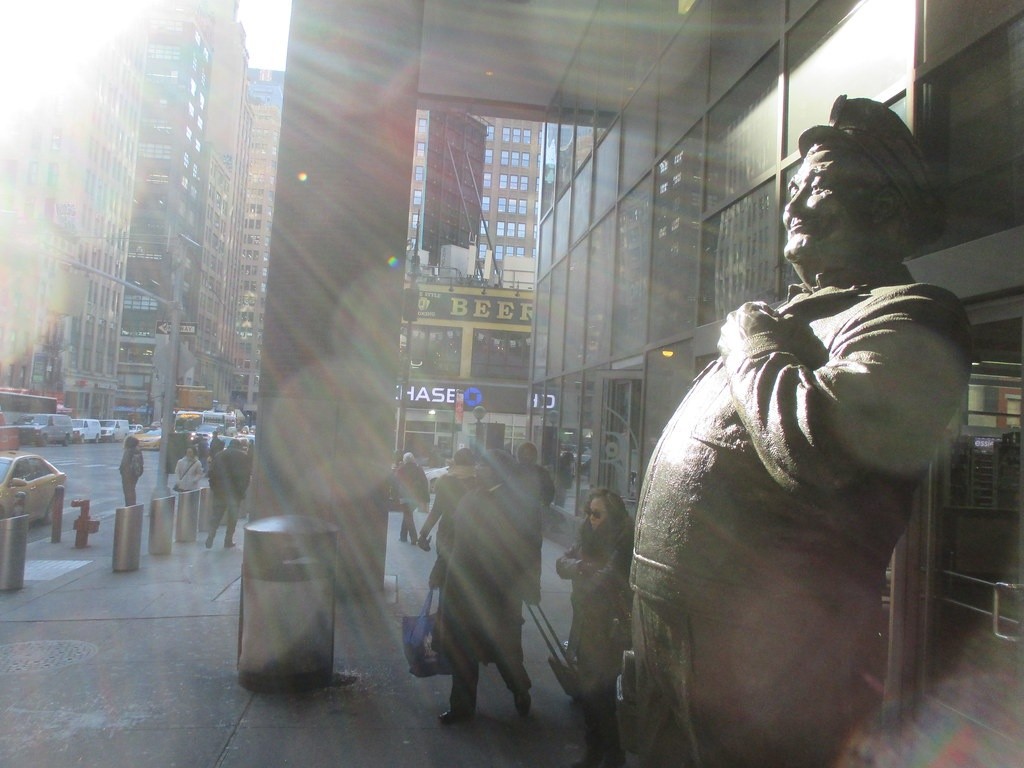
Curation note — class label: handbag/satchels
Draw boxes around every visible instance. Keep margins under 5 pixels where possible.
[172,483,179,492]
[399,587,455,677]
[416,462,431,503]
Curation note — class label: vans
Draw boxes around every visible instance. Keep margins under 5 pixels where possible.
[69,419,101,443]
[98,420,129,442]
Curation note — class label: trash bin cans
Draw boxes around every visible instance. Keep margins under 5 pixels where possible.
[237,513,340,696]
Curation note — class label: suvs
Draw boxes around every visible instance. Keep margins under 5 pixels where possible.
[13,414,72,447]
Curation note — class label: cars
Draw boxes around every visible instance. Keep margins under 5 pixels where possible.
[0,451,67,525]
[191,423,224,443]
[133,426,176,450]
[425,466,450,493]
[128,424,143,434]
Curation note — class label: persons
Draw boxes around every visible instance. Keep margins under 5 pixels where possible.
[619,92,980,766]
[174,426,259,549]
[393,451,431,545]
[517,441,557,507]
[556,488,636,768]
[428,448,547,724]
[119,436,144,507]
[418,448,479,588]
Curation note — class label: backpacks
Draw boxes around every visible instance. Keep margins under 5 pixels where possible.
[127,448,144,477]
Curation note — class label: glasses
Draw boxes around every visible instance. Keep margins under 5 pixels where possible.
[584,505,601,519]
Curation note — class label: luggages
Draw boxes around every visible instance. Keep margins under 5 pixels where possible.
[521,599,578,697]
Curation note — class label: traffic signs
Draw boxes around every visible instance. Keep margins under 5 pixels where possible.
[155,320,196,335]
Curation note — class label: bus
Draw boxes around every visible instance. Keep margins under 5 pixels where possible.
[174,410,236,436]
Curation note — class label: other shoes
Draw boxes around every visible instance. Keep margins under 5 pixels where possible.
[205,528,216,548]
[224,542,237,548]
[595,751,627,768]
[438,707,475,723]
[570,748,601,768]
[513,688,531,718]
